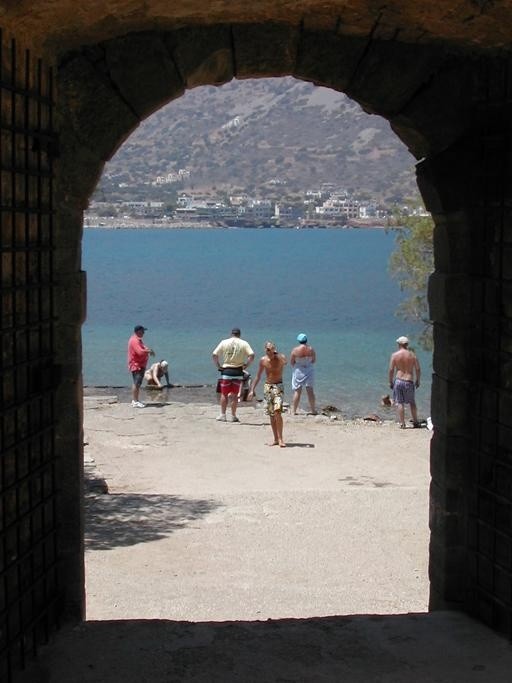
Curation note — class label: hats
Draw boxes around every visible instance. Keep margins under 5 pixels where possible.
[135,325,147,331]
[161,360,168,373]
[297,334,308,342]
[396,336,408,345]
[231,328,240,334]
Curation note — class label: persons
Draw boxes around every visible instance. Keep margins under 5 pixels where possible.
[290,333,318,415]
[388,335,421,428]
[211,327,255,422]
[380,393,400,408]
[247,340,289,447]
[237,363,256,403]
[144,358,172,389]
[128,325,155,408]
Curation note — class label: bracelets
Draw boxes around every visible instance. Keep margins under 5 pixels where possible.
[273,351,277,354]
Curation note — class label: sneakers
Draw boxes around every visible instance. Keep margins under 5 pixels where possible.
[400,424,405,429]
[131,400,146,408]
[414,422,421,427]
[232,417,239,422]
[216,416,226,421]
